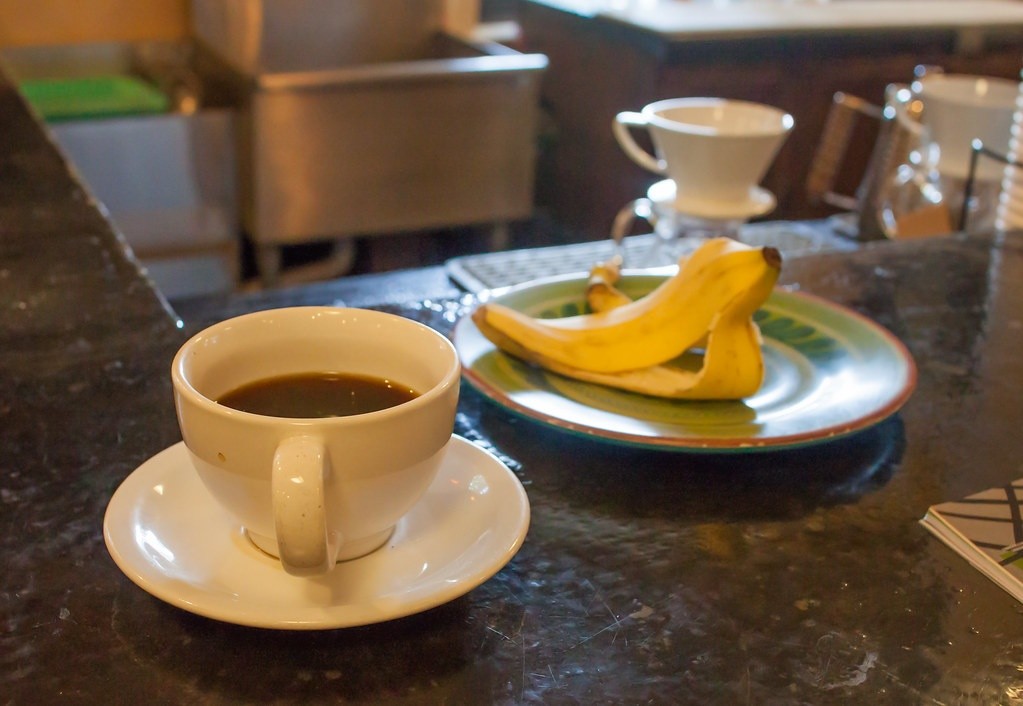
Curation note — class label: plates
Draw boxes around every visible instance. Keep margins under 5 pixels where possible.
[455,269,918,454]
[645,173,778,220]
[102,424,533,630]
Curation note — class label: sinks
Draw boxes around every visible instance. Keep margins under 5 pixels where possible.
[188,24,550,246]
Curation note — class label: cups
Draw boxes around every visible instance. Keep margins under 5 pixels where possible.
[884,74,1023,178]
[171,306,461,577]
[611,96,796,205]
